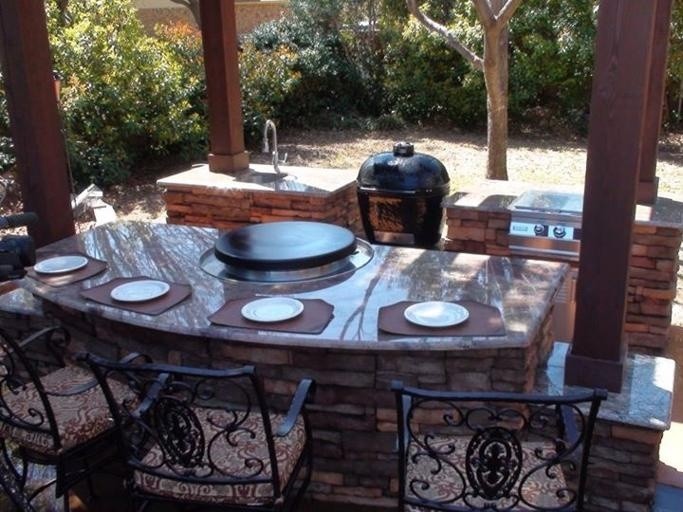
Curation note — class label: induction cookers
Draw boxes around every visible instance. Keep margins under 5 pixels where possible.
[508,191,585,245]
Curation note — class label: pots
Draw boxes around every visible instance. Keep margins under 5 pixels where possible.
[354,142,451,247]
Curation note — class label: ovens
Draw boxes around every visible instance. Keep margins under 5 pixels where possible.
[506,236,581,344]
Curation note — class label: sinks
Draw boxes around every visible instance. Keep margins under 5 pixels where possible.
[234,171,289,185]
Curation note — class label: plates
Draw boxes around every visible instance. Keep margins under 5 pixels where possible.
[109,280,171,302]
[404,300,469,328]
[35,255,88,274]
[239,299,304,324]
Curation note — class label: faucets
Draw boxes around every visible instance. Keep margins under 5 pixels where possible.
[261,120,288,170]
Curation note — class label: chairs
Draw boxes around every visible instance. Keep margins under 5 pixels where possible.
[0,324,155,512]
[387,379,609,512]
[81,350,319,512]
